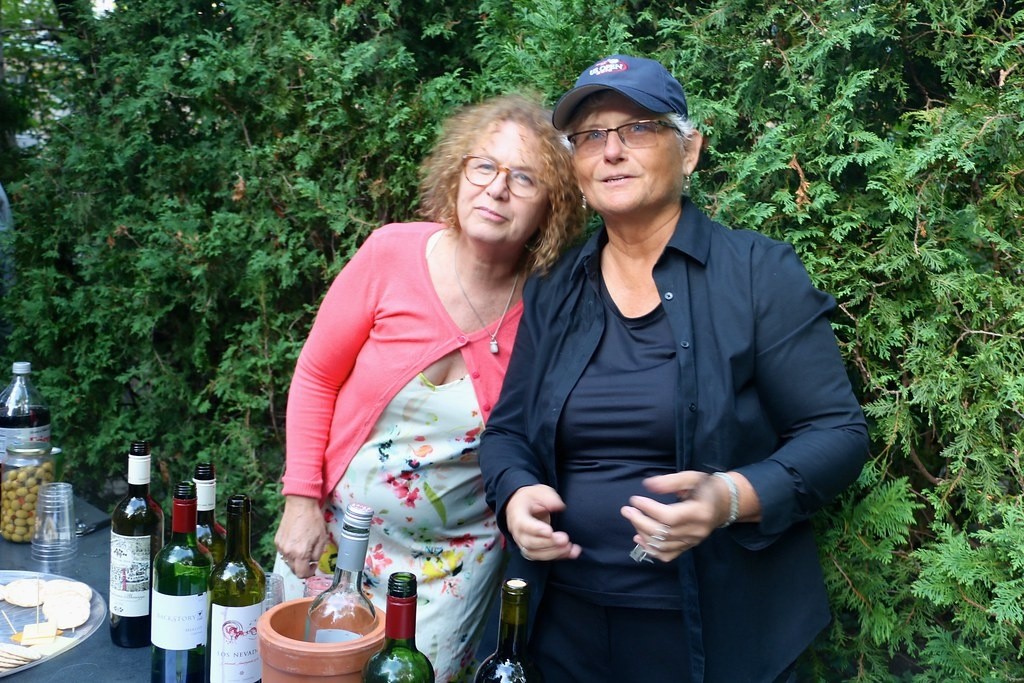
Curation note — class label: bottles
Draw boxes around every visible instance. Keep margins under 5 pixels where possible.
[109,441,165,648]
[191,462,227,567]
[304,503,379,643]
[0,441,56,542]
[474,578,531,683]
[152,481,213,683]
[0,362,56,486]
[363,572,435,683]
[208,495,267,683]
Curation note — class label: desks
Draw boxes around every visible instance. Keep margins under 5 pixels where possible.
[1,494,152,683]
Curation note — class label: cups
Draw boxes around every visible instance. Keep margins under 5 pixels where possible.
[304,574,334,596]
[32,483,78,563]
[265,571,285,612]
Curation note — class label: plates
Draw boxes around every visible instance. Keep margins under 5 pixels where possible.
[0,570,108,677]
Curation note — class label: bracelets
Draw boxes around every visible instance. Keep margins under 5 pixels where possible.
[713,471,739,528]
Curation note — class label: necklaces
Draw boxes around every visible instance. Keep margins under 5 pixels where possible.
[454,247,519,355]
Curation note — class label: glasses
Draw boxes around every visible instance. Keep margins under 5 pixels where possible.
[461,153,544,200]
[567,120,681,159]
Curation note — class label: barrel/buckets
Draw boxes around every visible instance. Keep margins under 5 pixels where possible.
[257,595,386,683]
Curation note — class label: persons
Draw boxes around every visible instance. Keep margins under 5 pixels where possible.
[478,55,871,683]
[268,94,588,683]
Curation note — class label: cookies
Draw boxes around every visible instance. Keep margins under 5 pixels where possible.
[0,576,93,674]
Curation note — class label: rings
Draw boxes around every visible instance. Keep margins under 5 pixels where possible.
[280,556,288,562]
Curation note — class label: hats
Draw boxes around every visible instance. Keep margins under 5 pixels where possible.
[552,56,688,131]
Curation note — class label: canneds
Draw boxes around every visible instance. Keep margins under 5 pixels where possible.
[0,441,64,543]
